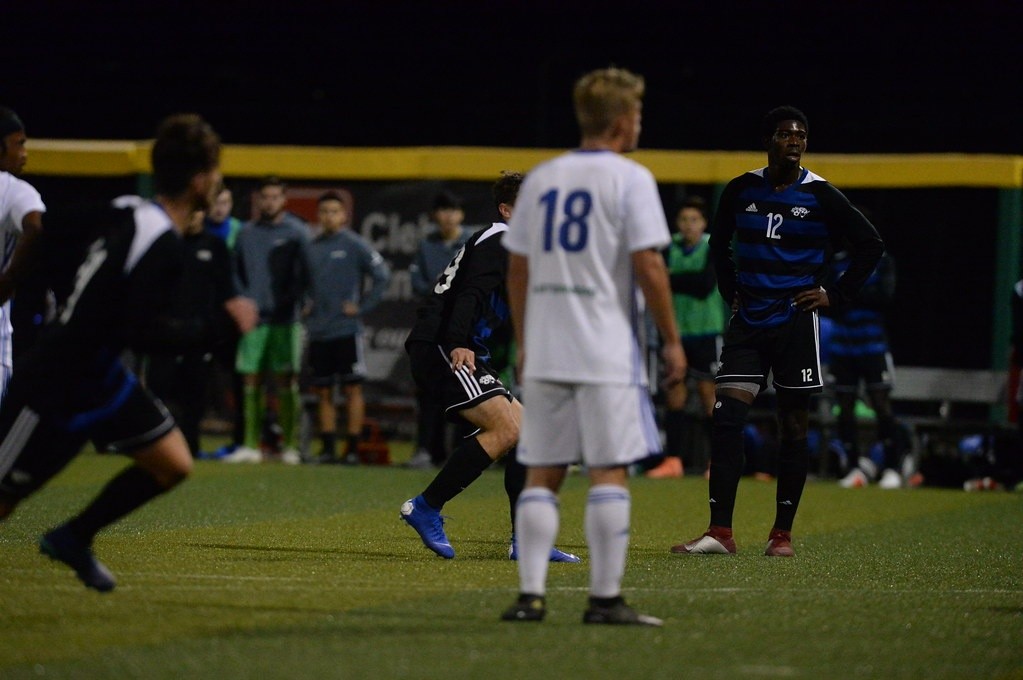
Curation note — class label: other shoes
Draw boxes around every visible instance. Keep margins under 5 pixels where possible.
[879,470,902,488]
[282,449,299,465]
[223,446,261,464]
[39,529,117,591]
[648,456,685,477]
[838,469,870,487]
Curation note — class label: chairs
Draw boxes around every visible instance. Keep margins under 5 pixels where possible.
[299,352,1022,463]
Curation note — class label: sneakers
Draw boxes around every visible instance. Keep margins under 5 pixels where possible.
[501,600,546,620]
[508,543,581,562]
[670,533,736,555]
[399,495,455,559]
[765,531,794,557]
[585,603,664,627]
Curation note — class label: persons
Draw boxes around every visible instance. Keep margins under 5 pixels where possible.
[671,107,883,557]
[402,173,577,564]
[0,104,221,593]
[0,178,913,489]
[498,69,683,626]
